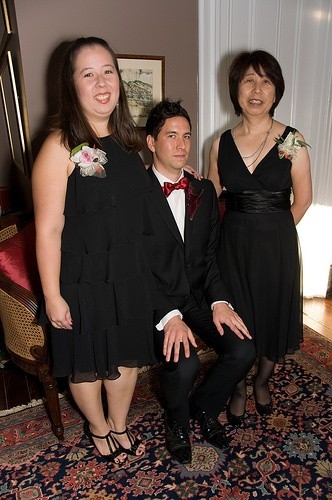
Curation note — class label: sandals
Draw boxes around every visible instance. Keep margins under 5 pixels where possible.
[83,421,145,463]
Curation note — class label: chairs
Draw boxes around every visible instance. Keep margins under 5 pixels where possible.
[0,211,208,441]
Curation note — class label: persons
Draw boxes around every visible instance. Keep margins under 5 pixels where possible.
[207,51,313,426]
[143,99,257,465]
[31,37,203,466]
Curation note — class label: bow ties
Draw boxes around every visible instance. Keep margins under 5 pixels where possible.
[161,178,190,197]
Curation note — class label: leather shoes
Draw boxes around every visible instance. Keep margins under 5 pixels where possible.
[164,409,192,465]
[197,408,229,448]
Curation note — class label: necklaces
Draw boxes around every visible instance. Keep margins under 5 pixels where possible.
[233,119,273,168]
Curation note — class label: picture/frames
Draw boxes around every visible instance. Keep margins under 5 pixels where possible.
[0,50,30,181]
[0,0,12,61]
[114,53,165,131]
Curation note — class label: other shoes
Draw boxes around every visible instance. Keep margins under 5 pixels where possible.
[252,377,272,416]
[225,389,248,428]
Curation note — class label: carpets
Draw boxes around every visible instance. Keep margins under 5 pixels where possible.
[1,323,332,500]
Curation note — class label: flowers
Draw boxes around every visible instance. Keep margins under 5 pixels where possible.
[275,130,312,159]
[69,141,108,180]
